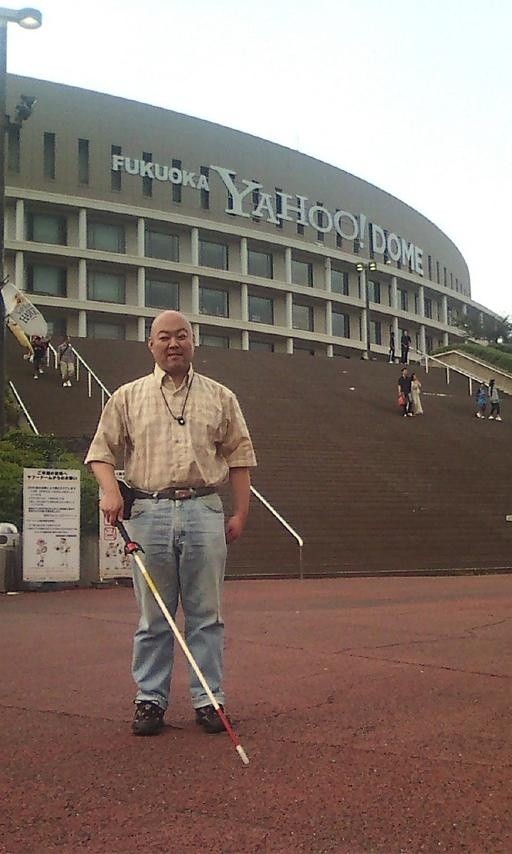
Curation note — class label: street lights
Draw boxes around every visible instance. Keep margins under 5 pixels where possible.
[357,262,376,360]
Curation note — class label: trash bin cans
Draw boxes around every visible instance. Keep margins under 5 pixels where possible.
[0,522,20,592]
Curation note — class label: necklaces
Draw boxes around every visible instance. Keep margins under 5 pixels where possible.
[159,374,195,424]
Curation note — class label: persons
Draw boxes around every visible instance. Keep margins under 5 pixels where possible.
[474,381,487,418]
[29,336,53,380]
[410,372,424,416]
[85,308,257,738]
[389,331,395,363]
[487,378,502,421]
[400,329,412,364]
[58,335,77,389]
[397,367,418,418]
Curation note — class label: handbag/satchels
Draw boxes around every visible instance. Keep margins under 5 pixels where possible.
[416,386,421,394]
[398,396,405,407]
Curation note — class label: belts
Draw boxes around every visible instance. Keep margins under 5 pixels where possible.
[131,487,221,502]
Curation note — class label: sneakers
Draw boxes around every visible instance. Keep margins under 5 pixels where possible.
[193,701,232,732]
[404,410,414,417]
[476,412,503,421]
[34,368,44,380]
[63,381,72,388]
[130,699,167,735]
